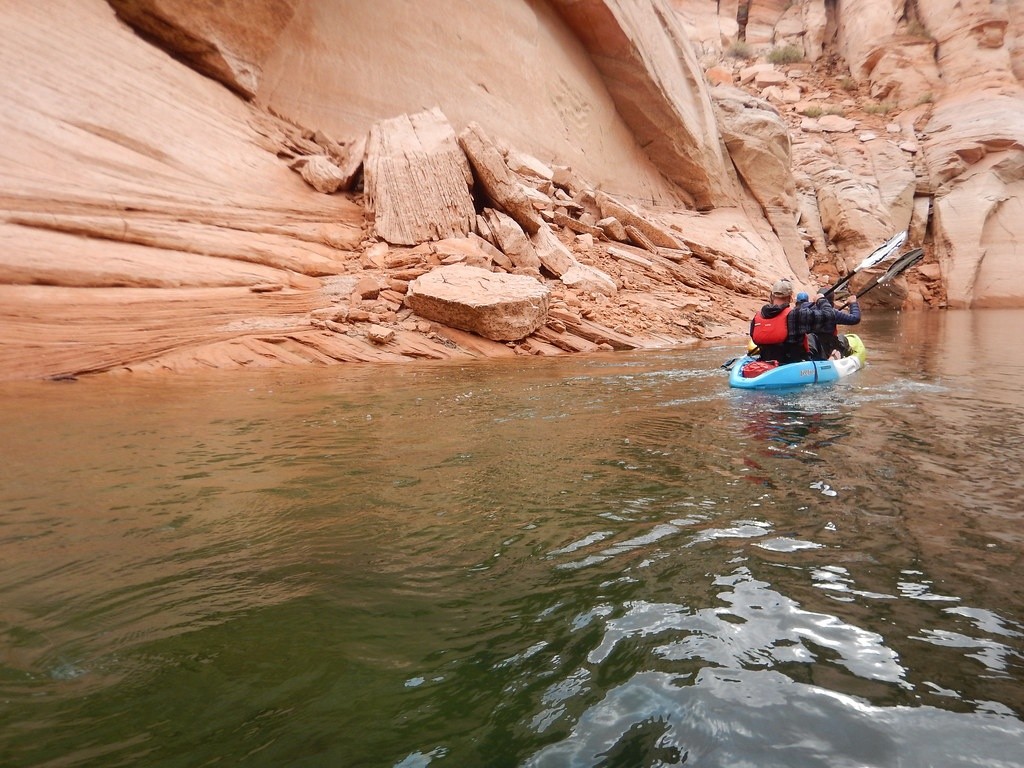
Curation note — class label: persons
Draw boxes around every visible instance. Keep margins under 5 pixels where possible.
[751,280,859,364]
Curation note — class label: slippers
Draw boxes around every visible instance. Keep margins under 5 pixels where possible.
[829,349,842,361]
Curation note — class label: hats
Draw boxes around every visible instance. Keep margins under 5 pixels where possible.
[797,293,809,303]
[772,280,793,297]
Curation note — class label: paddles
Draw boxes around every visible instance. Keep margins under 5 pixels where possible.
[725,230,907,370]
[840,247,925,313]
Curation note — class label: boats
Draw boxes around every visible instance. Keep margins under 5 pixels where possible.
[728,334,866,388]
